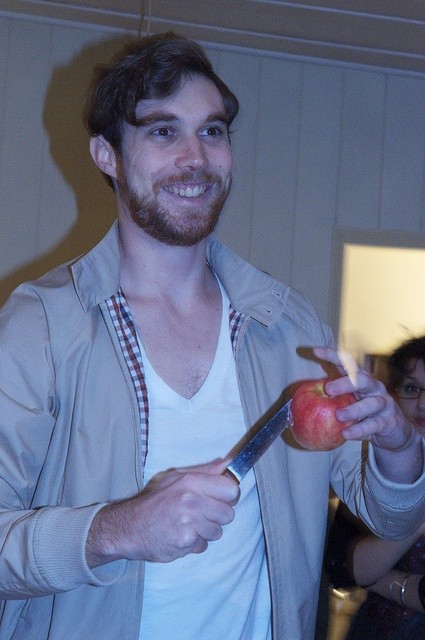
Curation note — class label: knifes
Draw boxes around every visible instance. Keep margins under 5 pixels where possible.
[220,398,293,486]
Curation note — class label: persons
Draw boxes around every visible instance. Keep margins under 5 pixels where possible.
[1,34,425,639]
[321,334,425,638]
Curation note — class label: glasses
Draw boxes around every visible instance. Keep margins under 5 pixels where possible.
[392,384,425,400]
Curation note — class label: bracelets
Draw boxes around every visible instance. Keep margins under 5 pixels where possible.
[387,571,414,618]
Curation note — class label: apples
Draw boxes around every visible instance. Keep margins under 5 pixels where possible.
[288,380,359,453]
